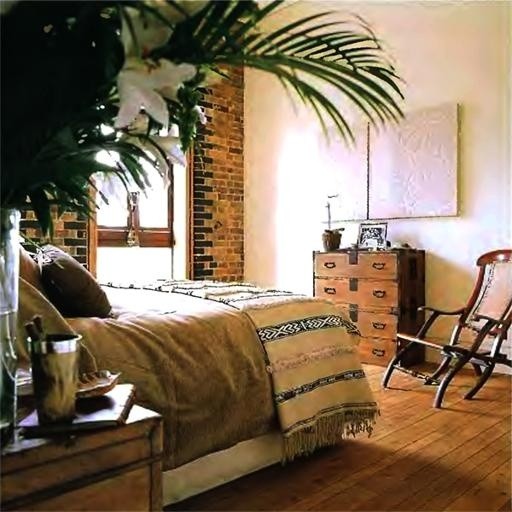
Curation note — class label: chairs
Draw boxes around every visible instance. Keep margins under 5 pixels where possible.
[382,249,511,409]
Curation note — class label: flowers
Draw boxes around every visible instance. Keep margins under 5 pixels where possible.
[0,0,409,258]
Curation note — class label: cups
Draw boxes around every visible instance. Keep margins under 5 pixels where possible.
[26,333,83,425]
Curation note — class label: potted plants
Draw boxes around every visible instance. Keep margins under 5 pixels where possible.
[320,229,346,249]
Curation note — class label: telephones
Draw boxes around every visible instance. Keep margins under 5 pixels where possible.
[14,371,33,397]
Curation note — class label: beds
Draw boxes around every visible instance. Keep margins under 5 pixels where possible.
[6,277,346,506]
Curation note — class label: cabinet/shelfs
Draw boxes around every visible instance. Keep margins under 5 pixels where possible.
[313,251,425,367]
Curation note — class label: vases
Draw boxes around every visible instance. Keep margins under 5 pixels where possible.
[1,208,20,429]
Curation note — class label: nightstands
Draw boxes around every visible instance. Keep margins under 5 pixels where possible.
[1,404,165,512]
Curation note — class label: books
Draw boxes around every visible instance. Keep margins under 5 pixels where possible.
[16,383,137,436]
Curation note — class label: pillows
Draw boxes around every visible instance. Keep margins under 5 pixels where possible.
[19,244,112,317]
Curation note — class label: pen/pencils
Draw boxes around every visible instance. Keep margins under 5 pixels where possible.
[24,314,45,341]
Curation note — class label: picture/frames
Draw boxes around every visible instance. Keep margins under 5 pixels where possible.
[356,222,388,251]
